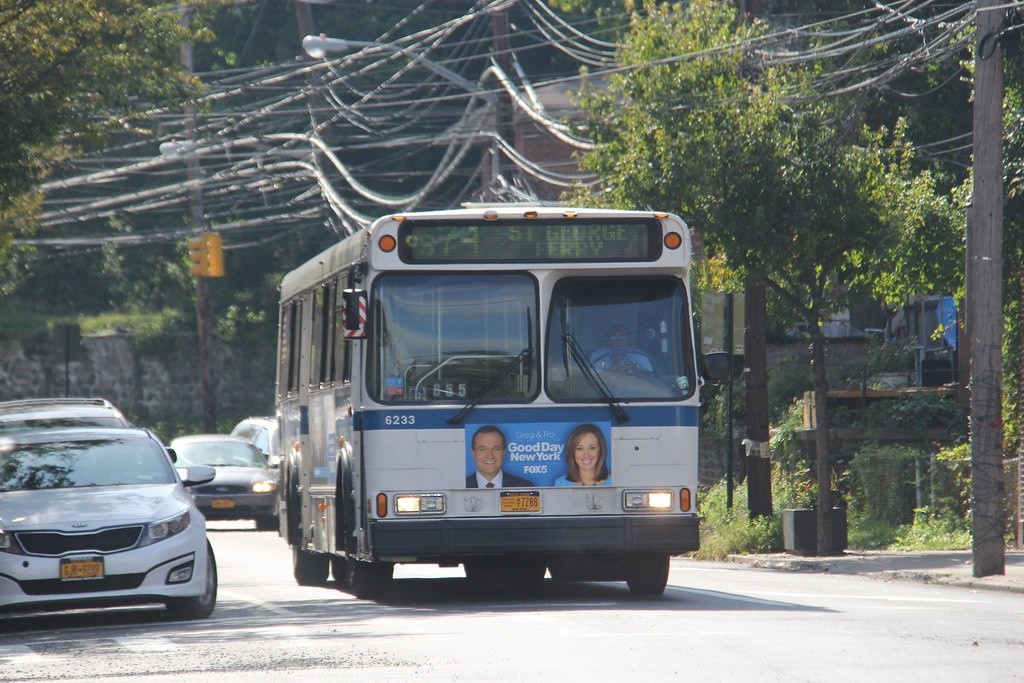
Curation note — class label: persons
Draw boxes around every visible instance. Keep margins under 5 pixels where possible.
[548,422,610,486]
[586,321,668,389]
[465,424,537,489]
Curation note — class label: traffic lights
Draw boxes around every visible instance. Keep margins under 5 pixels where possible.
[187,234,207,276]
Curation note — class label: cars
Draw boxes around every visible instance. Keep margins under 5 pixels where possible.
[0,424,222,629]
[168,436,283,530]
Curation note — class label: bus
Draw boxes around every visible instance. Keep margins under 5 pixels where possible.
[276,198,734,598]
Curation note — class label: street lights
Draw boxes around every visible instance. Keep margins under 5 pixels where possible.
[304,31,524,198]
